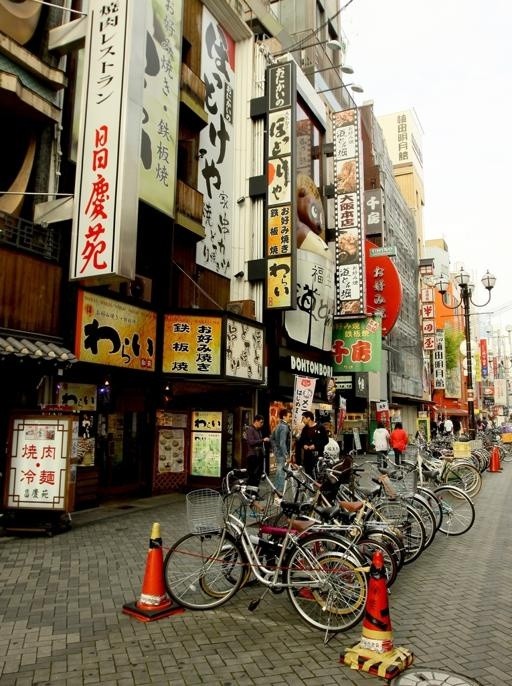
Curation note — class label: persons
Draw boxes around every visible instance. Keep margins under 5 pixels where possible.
[298,411,330,479]
[436,418,444,436]
[442,417,453,435]
[371,422,391,469]
[388,422,408,466]
[476,420,485,431]
[481,417,488,429]
[267,409,293,495]
[244,414,271,501]
[430,418,437,438]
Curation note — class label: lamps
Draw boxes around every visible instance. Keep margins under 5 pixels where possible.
[271,40,364,93]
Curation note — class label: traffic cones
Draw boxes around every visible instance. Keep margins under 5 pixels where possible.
[121,521,185,623]
[340,551,414,680]
[486,448,503,473]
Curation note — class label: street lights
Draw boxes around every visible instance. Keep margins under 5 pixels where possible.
[436,266,496,441]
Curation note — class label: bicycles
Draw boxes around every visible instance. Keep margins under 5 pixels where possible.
[397,427,512,499]
